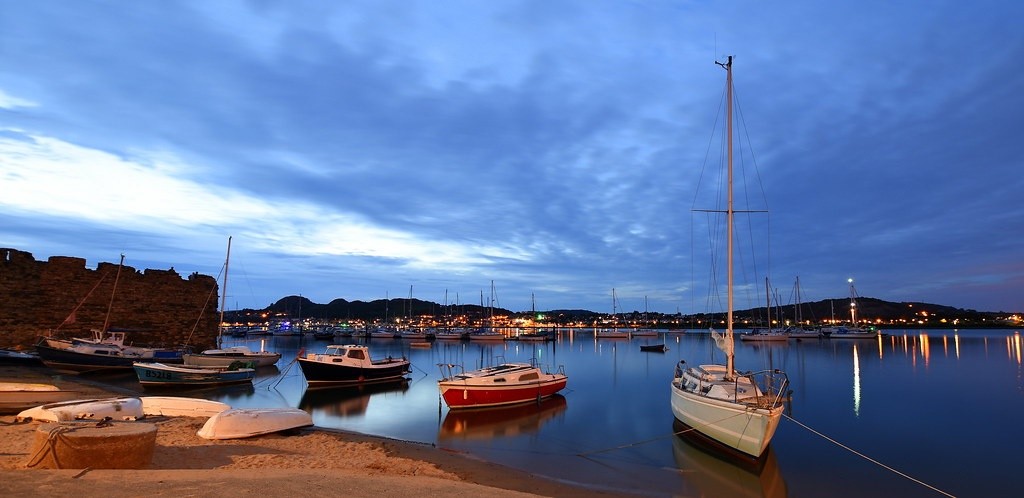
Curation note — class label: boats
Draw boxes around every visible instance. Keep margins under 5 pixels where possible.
[296,378,410,416]
[196,406,314,441]
[1,383,84,411]
[439,361,568,409]
[140,395,230,414]
[15,395,144,423]
[439,394,567,434]
[639,344,667,352]
[297,344,411,385]
[672,416,788,498]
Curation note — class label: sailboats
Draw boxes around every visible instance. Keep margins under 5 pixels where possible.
[670,54,786,458]
[740,276,878,341]
[32,235,281,386]
[595,288,658,338]
[223,279,558,339]
